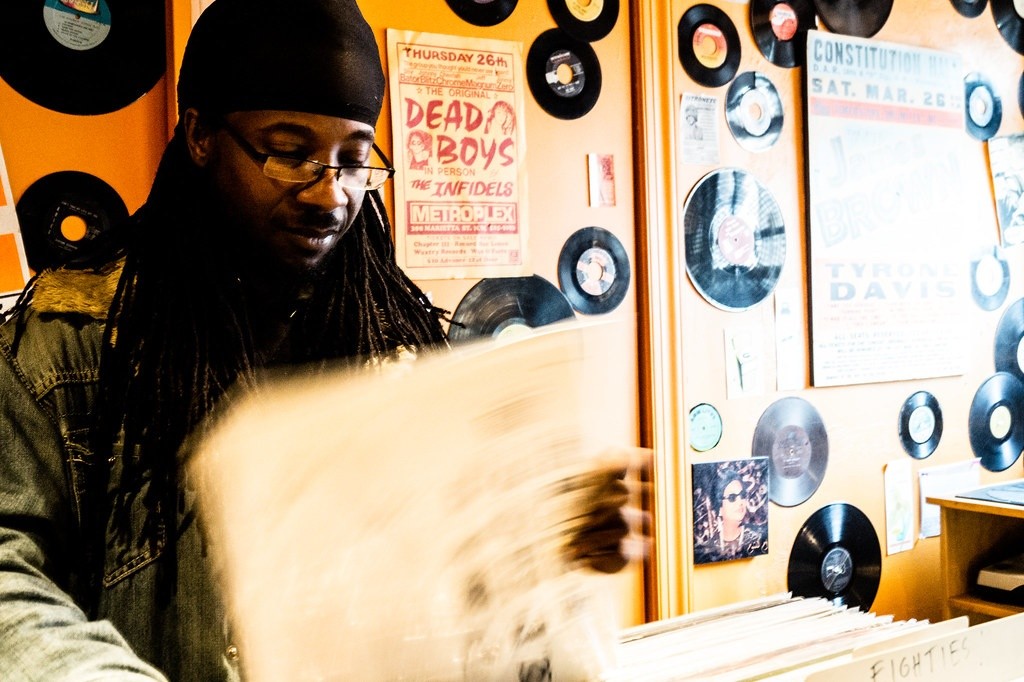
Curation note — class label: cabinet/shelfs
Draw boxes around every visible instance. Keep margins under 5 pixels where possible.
[926,478,1023,620]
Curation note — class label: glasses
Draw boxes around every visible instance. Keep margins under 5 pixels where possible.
[205,118,396,191]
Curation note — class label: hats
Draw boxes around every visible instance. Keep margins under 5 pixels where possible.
[178,0,385,125]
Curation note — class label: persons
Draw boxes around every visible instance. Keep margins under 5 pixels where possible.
[704,473,760,558]
[0,0,631,681]
